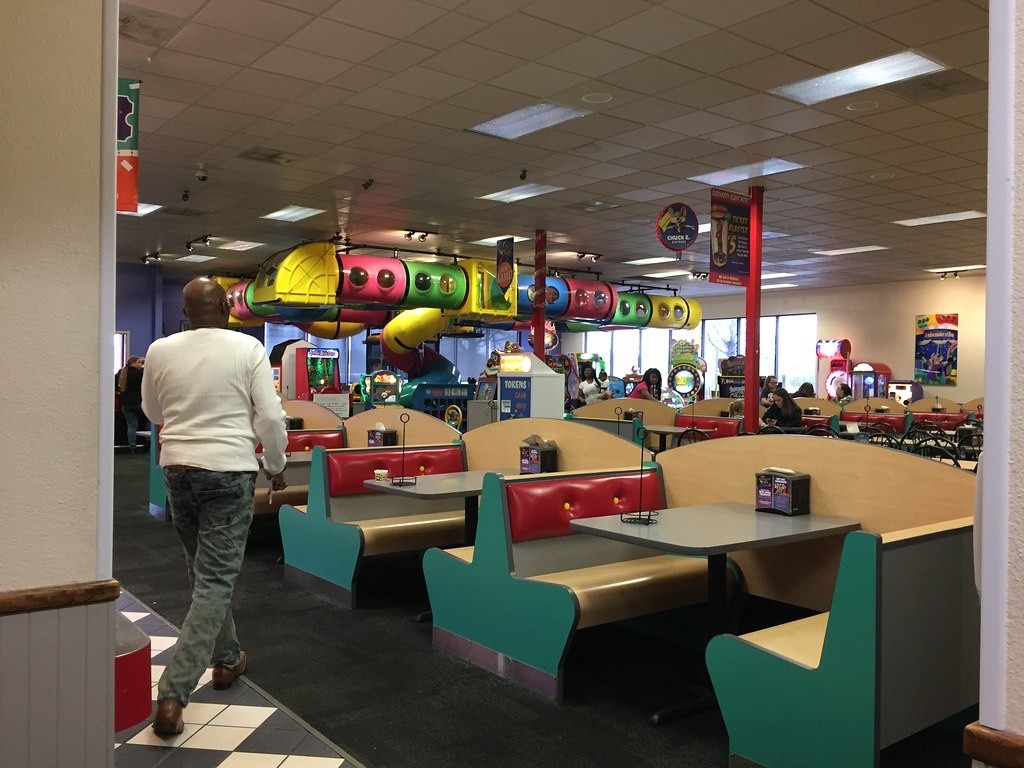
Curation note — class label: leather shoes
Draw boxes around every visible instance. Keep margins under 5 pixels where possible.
[153,700,184,733]
[212,651,246,691]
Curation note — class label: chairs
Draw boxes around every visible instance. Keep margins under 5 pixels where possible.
[760,419,983,473]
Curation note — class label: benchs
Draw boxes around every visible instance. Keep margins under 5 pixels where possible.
[150,421,172,522]
[252,425,347,522]
[841,411,915,440]
[650,412,745,453]
[703,517,980,768]
[424,461,744,702]
[279,439,468,611]
[801,415,841,438]
[565,413,645,447]
[905,408,984,433]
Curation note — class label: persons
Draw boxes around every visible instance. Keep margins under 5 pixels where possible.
[791,382,817,399]
[578,367,612,407]
[762,388,802,427]
[141,275,289,741]
[727,398,768,435]
[828,384,856,409]
[595,371,609,400]
[760,376,778,407]
[115,356,144,454]
[626,368,667,405]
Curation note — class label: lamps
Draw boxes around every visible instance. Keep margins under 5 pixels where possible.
[576,253,585,262]
[690,272,708,280]
[592,255,600,263]
[418,233,429,241]
[519,170,527,181]
[142,258,149,266]
[361,180,373,190]
[181,191,189,201]
[154,254,161,261]
[203,236,210,245]
[404,230,415,241]
[185,242,194,252]
[939,273,959,281]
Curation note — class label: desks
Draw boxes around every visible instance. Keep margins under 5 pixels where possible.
[363,470,547,621]
[838,421,864,427]
[933,457,977,472]
[568,505,862,730]
[253,451,313,466]
[645,426,716,453]
[927,430,955,435]
[870,435,960,448]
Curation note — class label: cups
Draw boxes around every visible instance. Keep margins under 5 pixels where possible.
[374,470,388,483]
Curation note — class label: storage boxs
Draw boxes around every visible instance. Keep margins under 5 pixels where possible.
[624,411,643,426]
[804,408,821,415]
[876,409,890,413]
[755,470,810,517]
[518,446,557,475]
[932,408,946,413]
[286,418,304,430]
[367,429,397,446]
